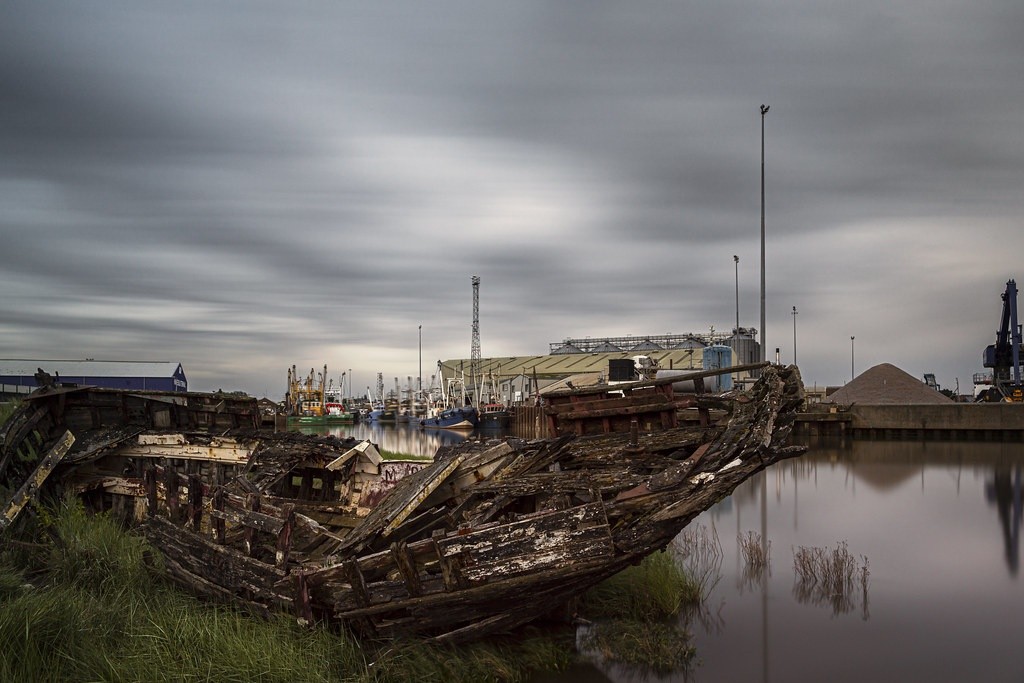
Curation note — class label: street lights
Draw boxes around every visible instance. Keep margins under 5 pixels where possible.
[791,305,798,365]
[734,253,743,382]
[418,325,423,392]
[850,335,855,381]
[760,101,774,363]
